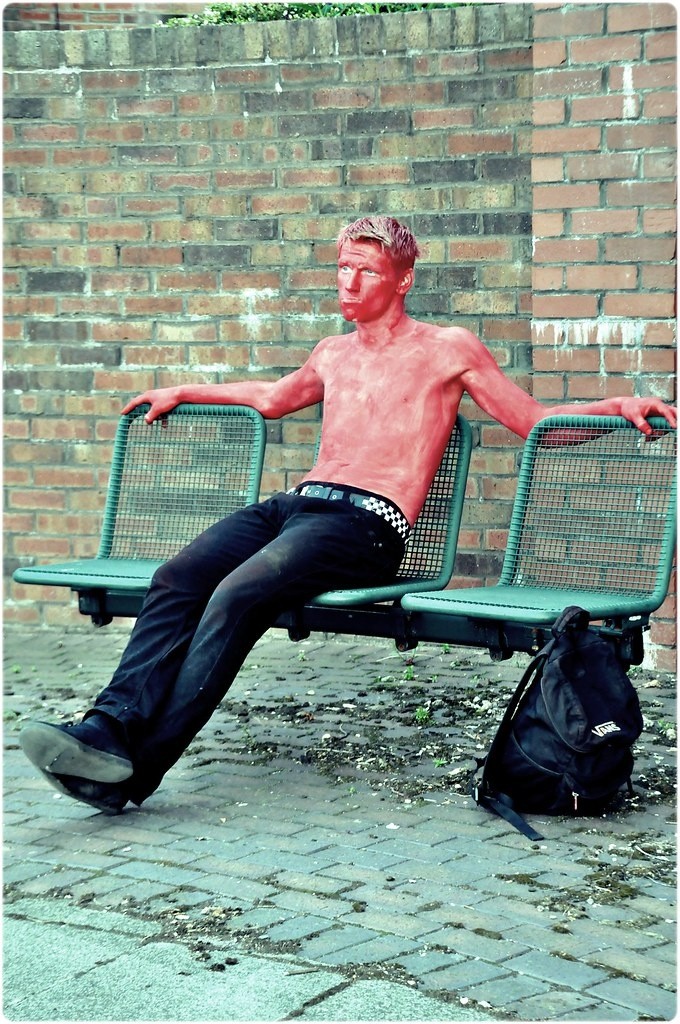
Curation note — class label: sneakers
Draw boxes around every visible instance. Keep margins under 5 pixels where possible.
[18,710,134,784]
[41,771,129,814]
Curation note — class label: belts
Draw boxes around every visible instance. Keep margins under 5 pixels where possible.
[285,488,412,541]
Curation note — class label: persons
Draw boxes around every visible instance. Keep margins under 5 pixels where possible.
[18,211,679,816]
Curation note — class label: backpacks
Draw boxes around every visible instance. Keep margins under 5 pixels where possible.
[468,606,646,841]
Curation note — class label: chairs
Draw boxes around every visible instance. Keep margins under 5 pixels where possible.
[310,417,476,607]
[10,398,270,596]
[403,410,676,623]
